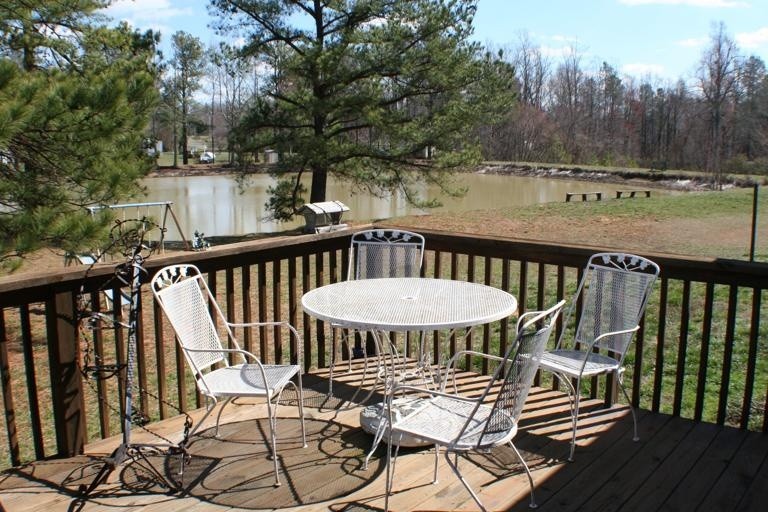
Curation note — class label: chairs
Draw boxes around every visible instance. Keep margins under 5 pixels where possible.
[328,228,440,409]
[151,264,309,487]
[364,299,566,512]
[499,253,661,462]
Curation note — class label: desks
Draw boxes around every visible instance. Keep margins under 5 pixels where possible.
[301,277,518,470]
